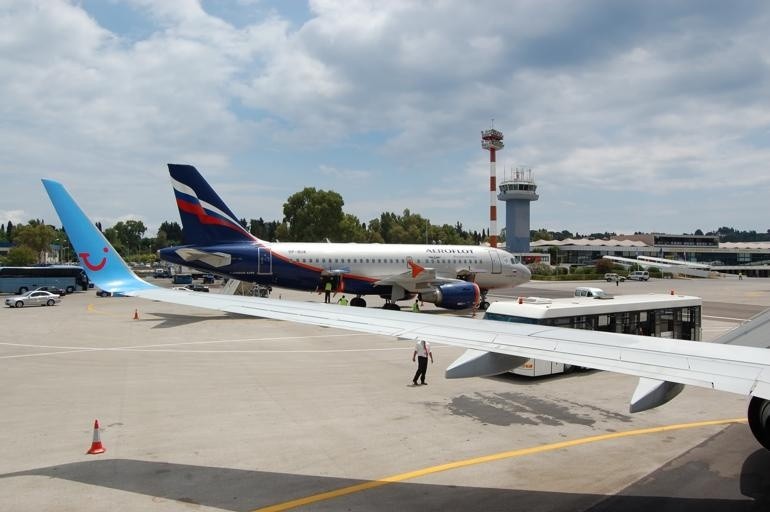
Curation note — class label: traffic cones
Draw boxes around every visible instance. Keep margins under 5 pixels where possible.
[130,310,141,319]
[87,421,105,453]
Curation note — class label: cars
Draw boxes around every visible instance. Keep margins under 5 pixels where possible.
[5,287,66,308]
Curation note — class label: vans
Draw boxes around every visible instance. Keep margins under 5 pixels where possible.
[629,271,649,280]
[574,285,604,298]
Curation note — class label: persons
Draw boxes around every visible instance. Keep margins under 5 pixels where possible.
[616,276,619,286]
[337,296,348,305]
[325,282,332,305]
[413,299,420,314]
[413,340,434,385]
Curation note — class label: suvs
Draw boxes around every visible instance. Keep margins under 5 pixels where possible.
[605,273,626,282]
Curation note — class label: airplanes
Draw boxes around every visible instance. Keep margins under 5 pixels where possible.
[151,161,534,312]
[39,177,770,448]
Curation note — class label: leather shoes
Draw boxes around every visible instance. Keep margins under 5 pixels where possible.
[413,379,427,385]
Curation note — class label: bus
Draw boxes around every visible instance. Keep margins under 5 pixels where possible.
[1,267,88,294]
[484,293,701,378]
